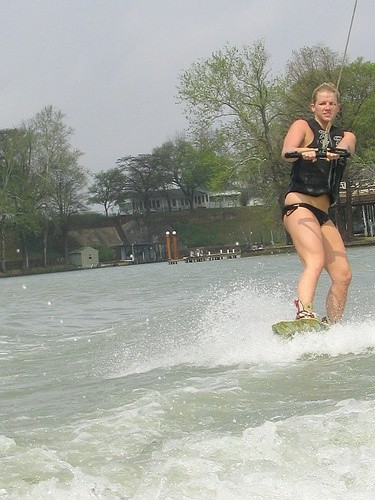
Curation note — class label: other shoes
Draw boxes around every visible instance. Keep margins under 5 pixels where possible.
[322,315,333,324]
[294,298,316,319]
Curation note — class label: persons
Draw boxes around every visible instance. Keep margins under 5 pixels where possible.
[281,82,356,326]
